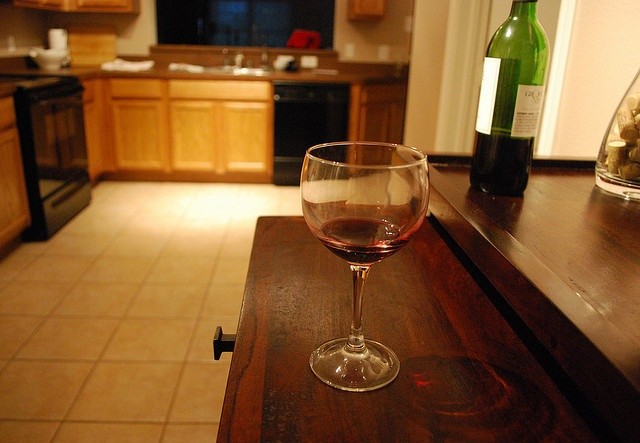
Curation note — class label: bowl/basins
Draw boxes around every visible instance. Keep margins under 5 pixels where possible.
[62,56,72,67]
[273,59,288,72]
[27,48,67,72]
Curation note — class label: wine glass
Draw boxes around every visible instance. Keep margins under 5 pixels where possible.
[300,143,430,393]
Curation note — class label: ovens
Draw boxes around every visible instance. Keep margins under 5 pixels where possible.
[273,81,351,186]
[13,76,92,242]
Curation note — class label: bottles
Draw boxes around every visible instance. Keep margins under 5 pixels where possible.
[468,0,551,197]
[594,68,640,201]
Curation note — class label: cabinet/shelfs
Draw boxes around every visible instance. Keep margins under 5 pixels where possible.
[80,77,105,185]
[70,1,139,15]
[105,76,171,181]
[1,96,31,262]
[168,80,274,185]
[11,1,69,12]
[348,78,407,178]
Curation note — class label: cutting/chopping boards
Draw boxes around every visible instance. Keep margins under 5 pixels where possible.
[69,23,117,68]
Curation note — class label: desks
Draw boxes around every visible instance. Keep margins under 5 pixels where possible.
[392,147,640,443]
[212,215,608,443]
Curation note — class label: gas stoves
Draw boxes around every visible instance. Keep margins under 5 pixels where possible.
[1,71,83,102]
